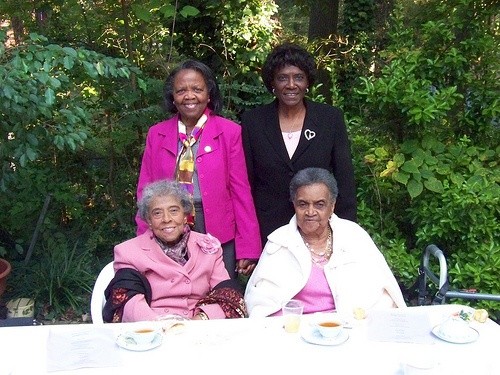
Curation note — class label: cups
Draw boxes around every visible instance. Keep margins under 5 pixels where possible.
[124,328,157,345]
[317,319,343,338]
[281,300,304,333]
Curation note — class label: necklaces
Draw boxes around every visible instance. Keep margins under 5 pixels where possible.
[300,227,337,263]
[277,105,305,139]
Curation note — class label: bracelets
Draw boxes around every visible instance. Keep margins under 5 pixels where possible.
[196,311,210,322]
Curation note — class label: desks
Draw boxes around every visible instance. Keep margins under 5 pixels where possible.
[0,304,500,375]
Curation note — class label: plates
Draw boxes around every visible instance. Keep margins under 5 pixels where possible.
[300,322,350,346]
[429,319,480,345]
[116,331,163,351]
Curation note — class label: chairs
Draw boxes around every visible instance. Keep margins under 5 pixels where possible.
[90,259,115,323]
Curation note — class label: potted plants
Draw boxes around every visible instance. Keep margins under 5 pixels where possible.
[2,258,11,303]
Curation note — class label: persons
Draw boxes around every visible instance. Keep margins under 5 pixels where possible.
[235,168,409,319]
[134,61,262,291]
[102,180,248,322]
[240,43,358,276]
